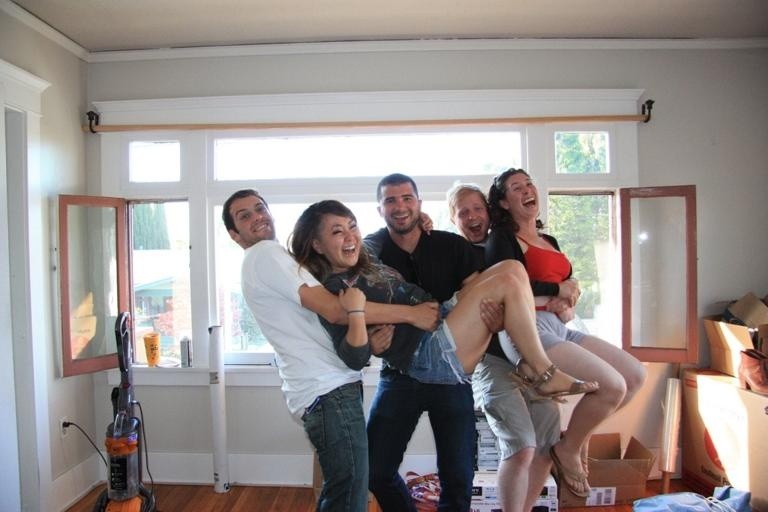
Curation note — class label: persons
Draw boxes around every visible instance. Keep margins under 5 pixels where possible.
[447,183,575,511]
[366,173,505,512]
[486,168,646,497]
[223,189,442,512]
[291,199,599,405]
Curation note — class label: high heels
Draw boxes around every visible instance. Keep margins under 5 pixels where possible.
[524,364,600,401]
[508,359,567,403]
[739,350,768,396]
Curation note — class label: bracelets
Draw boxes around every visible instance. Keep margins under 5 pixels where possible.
[347,309,366,315]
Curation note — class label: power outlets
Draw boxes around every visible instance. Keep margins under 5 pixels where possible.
[58,413,74,440]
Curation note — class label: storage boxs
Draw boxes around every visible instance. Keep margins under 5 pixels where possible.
[681,367,768,512]
[469,469,558,512]
[557,432,658,509]
[701,313,768,380]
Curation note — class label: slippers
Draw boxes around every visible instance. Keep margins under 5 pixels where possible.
[548,446,591,497]
[560,431,589,478]
